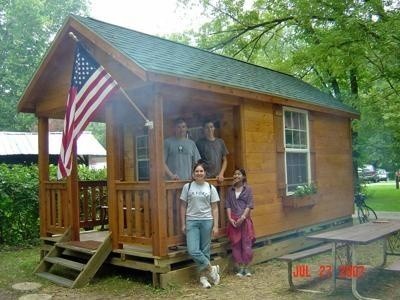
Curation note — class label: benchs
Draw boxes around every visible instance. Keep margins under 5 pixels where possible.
[275,242,346,296]
[384,260,400,273]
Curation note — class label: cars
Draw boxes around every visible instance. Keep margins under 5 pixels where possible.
[357,164,388,183]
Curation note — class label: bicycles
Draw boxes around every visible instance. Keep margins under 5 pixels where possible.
[355,193,377,224]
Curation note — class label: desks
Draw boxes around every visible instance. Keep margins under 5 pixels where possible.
[304,219,400,300]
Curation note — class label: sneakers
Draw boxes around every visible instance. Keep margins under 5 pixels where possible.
[209,265,220,285]
[234,267,252,278]
[199,276,211,289]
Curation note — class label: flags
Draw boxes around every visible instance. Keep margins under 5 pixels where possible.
[54,39,122,182]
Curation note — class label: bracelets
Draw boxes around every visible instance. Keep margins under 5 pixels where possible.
[240,216,246,222]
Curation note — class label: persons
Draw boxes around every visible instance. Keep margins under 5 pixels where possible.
[161,115,205,181]
[179,161,222,289]
[194,120,231,184]
[223,168,256,277]
